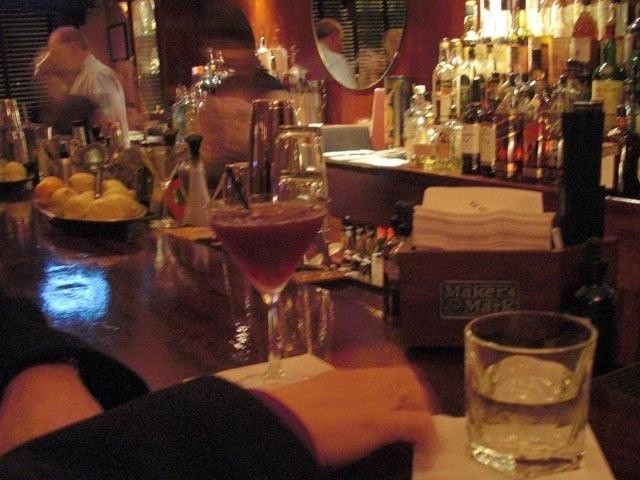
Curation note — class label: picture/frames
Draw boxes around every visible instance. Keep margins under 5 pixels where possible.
[107,23,129,62]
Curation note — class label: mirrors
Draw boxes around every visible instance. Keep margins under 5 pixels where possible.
[311,0,407,91]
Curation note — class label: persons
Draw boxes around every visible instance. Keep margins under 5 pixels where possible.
[315,18,358,90]
[0,337,439,480]
[29,25,132,150]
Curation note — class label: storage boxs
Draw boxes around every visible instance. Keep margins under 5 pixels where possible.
[396,229,566,347]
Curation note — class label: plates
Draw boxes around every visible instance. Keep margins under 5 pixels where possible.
[33,195,149,232]
[1,172,38,194]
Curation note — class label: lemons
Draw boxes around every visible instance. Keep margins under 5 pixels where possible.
[6,162,26,180]
[39,173,138,219]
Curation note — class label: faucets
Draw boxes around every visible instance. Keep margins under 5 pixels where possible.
[194,61,224,97]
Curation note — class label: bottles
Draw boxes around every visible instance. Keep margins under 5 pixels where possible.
[182,136,213,229]
[258,37,329,124]
[369,74,410,151]
[404,0,640,201]
[159,128,179,179]
[69,118,126,164]
[344,213,412,322]
[563,260,622,372]
[248,99,298,199]
[173,50,232,128]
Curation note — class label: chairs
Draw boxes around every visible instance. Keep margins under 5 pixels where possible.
[222,99,270,199]
[270,100,297,137]
[370,87,385,149]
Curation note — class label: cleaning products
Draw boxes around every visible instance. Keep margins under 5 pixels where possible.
[162,134,210,228]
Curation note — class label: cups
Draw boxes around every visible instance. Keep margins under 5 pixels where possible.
[463,310,599,479]
[271,125,328,201]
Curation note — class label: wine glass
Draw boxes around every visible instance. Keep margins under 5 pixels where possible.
[208,200,330,390]
